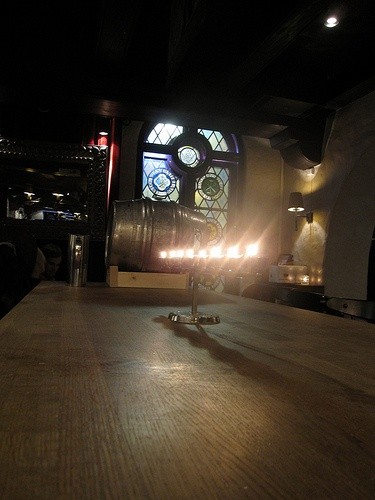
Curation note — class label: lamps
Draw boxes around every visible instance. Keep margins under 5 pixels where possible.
[287,192,313,231]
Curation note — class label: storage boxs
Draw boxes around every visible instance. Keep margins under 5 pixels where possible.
[269,264,307,284]
